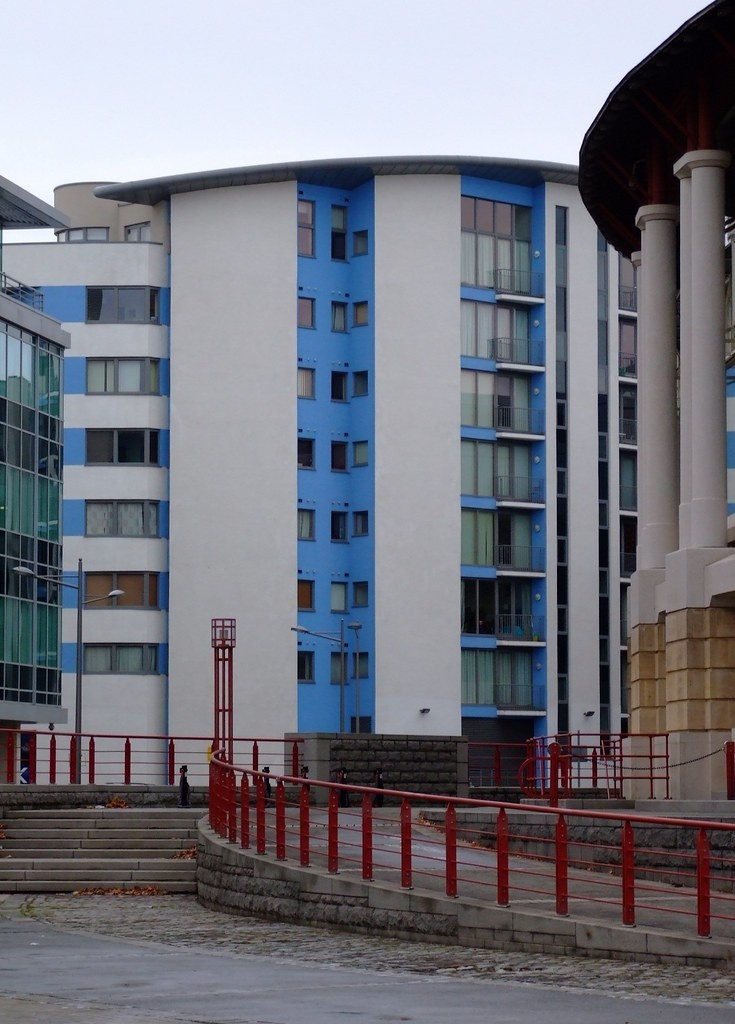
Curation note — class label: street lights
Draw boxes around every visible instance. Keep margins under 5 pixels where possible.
[12,565,125,784]
[290,626,345,733]
[346,622,363,733]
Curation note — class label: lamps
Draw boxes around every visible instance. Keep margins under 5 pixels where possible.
[584,711,594,716]
[420,708,431,714]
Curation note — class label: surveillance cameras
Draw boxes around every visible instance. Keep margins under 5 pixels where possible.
[49,723,55,730]
